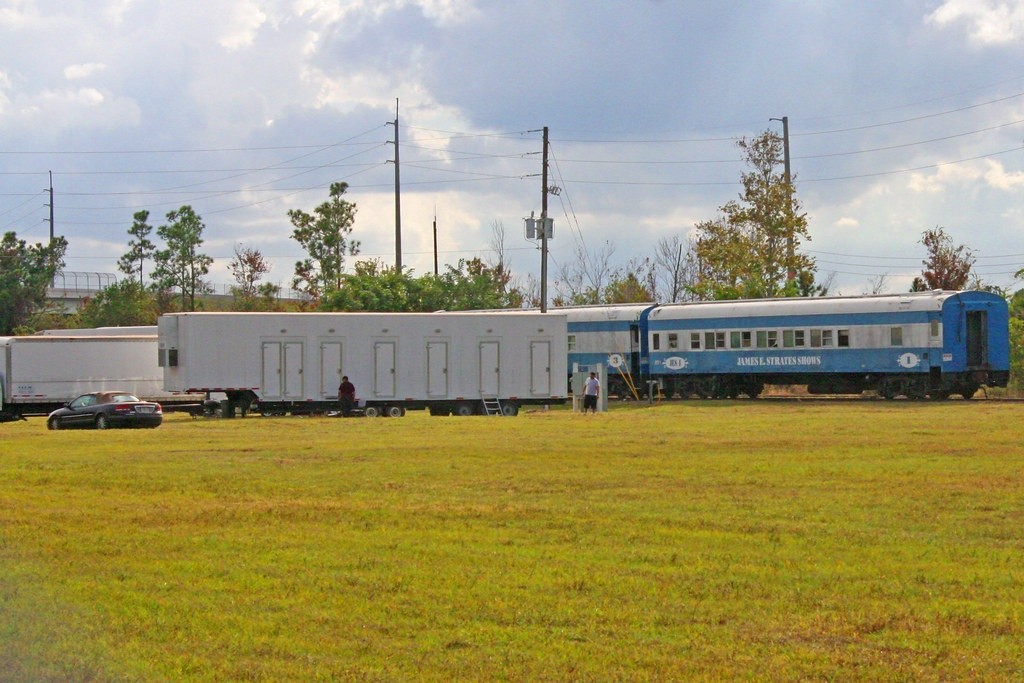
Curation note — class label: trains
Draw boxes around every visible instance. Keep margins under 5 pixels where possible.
[564,288,1011,402]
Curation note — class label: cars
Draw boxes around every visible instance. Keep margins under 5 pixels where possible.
[46,390,163,431]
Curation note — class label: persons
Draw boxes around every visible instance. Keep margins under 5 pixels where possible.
[338,376,356,418]
[581,373,600,416]
